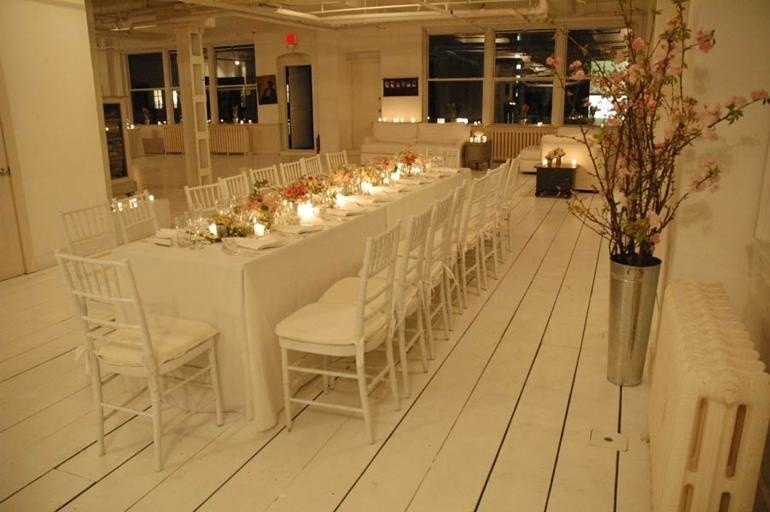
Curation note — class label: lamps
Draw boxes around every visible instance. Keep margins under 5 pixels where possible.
[284,34,297,49]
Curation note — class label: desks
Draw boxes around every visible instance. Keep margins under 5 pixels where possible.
[465,141,491,170]
[113,163,468,430]
[534,161,576,199]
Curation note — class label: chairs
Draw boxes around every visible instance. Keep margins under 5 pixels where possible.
[113,190,161,245]
[273,219,404,444]
[183,180,227,213]
[280,150,348,185]
[393,155,520,332]
[249,164,279,190]
[53,248,224,473]
[357,190,454,360]
[319,204,435,399]
[216,171,250,201]
[58,199,119,311]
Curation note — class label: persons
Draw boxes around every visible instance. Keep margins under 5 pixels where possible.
[141,104,157,124]
[260,80,276,104]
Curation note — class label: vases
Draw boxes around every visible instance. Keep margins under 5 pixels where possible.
[607,255,662,387]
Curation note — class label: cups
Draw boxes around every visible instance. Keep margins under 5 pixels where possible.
[172,152,451,257]
[112,186,156,214]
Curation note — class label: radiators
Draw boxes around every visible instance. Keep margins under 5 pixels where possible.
[163,126,249,156]
[485,125,559,161]
[651,279,770,512]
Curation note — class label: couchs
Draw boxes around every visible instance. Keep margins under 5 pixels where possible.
[362,122,470,167]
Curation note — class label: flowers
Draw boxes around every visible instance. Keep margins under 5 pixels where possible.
[543,0,770,266]
